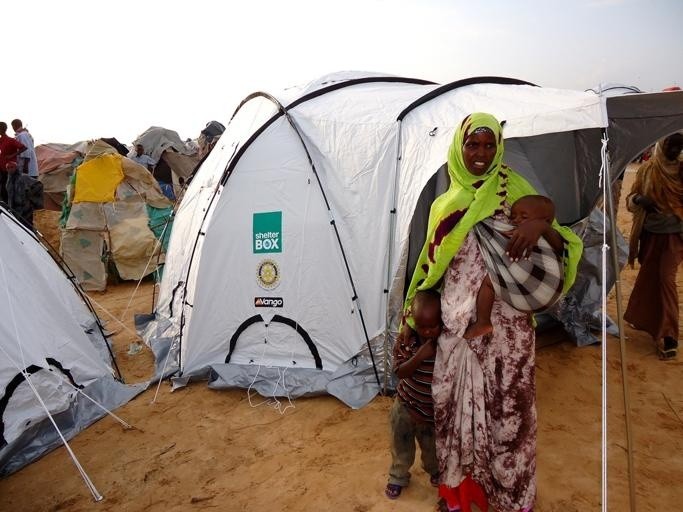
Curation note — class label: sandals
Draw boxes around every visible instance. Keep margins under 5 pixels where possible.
[385,483,401,498]
[430,472,440,488]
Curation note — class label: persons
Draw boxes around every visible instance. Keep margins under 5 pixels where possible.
[130,144,156,174]
[6,160,45,229]
[384,287,440,501]
[11,119,39,181]
[462,194,565,341]
[391,111,584,511]
[622,134,683,360]
[1,121,29,208]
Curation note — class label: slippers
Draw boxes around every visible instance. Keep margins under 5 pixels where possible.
[658,347,678,360]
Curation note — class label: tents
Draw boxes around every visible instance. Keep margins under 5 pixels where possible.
[0,204,150,484]
[585,80,645,96]
[133,71,682,412]
